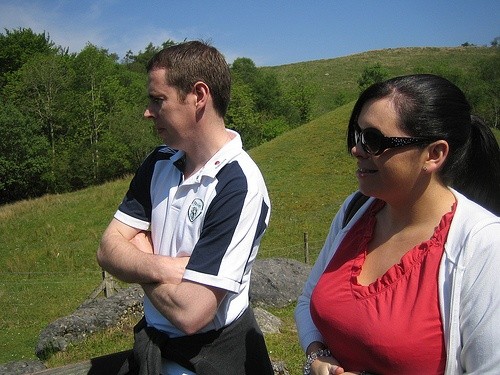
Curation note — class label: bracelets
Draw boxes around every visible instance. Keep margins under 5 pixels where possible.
[303,349,331,375]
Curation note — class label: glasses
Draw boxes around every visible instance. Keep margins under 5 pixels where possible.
[353,123,427,156]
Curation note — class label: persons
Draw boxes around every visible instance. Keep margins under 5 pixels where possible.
[96,40,276,375]
[295,74,500,375]
